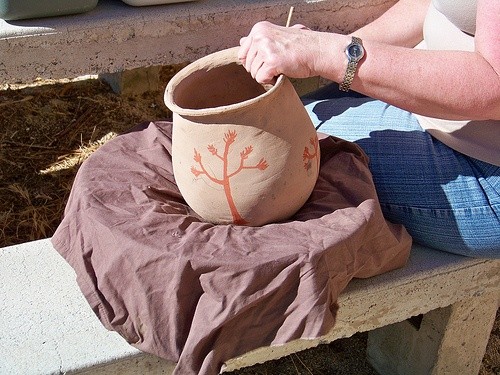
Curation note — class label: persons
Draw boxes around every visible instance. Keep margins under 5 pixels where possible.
[236,0,499,258]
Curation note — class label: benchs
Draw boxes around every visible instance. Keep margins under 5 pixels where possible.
[1,218,500,375]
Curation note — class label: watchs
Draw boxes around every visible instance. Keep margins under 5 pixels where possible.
[338,35,365,92]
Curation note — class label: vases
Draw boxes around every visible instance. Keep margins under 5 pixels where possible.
[164,44,322,227]
